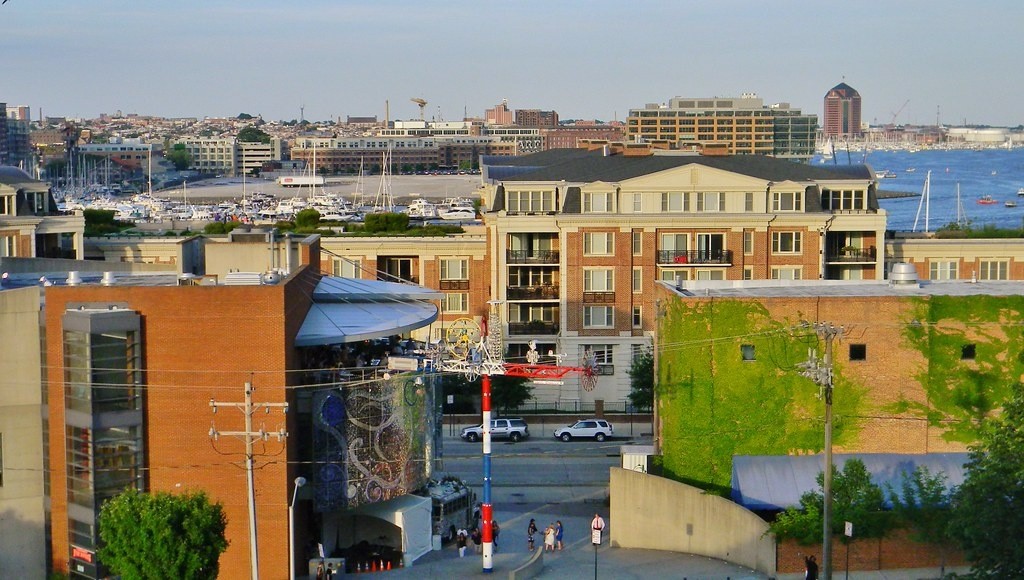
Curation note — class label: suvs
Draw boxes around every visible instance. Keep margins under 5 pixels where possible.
[553,419,614,442]
[459,416,530,442]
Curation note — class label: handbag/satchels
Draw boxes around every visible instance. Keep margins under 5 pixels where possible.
[528,537,534,542]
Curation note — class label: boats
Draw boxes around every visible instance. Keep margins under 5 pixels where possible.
[52,173,476,226]
[814,133,1024,207]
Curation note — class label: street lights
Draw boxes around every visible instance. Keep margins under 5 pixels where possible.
[289,476,307,580]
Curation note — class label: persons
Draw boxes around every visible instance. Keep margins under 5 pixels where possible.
[316,562,333,580]
[215,212,248,224]
[591,513,606,545]
[805,555,819,580]
[300,334,416,385]
[457,520,500,559]
[528,519,563,552]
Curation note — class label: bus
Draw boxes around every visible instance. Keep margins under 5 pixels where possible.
[417,480,482,543]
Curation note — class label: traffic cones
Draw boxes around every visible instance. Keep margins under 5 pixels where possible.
[399,560,403,568]
[365,563,368,573]
[380,559,384,571]
[357,563,361,573]
[372,562,376,572]
[387,561,391,570]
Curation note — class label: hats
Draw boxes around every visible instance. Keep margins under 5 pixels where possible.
[530,518,535,522]
